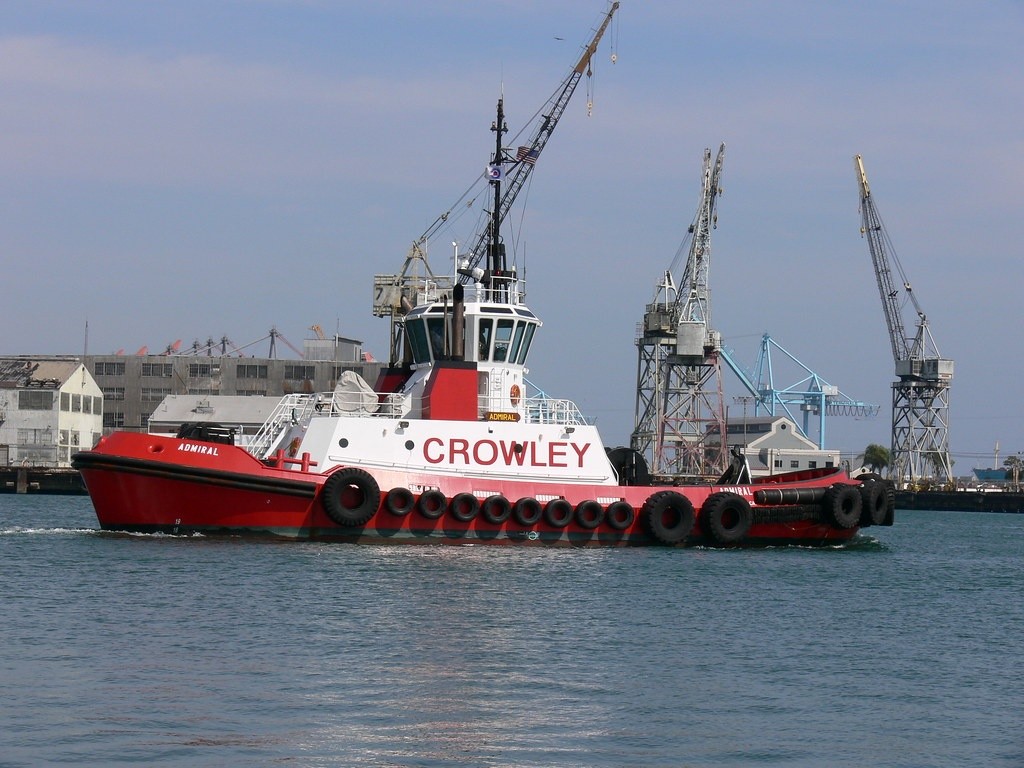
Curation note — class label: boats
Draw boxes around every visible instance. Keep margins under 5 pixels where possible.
[68,240,897,553]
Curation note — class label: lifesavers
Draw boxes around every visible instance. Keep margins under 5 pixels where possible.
[879,479,895,526]
[543,499,574,528]
[513,496,542,526]
[700,491,753,543]
[606,501,634,529]
[855,473,884,482]
[640,490,696,544]
[418,490,447,519]
[322,467,381,526]
[859,480,888,525]
[824,485,862,529]
[483,495,512,523]
[289,436,300,456]
[387,487,414,516]
[511,384,521,404]
[575,500,604,529]
[450,492,480,521]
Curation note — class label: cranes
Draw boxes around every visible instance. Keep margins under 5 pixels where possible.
[855,152,959,492]
[372,0,621,367]
[626,140,734,488]
